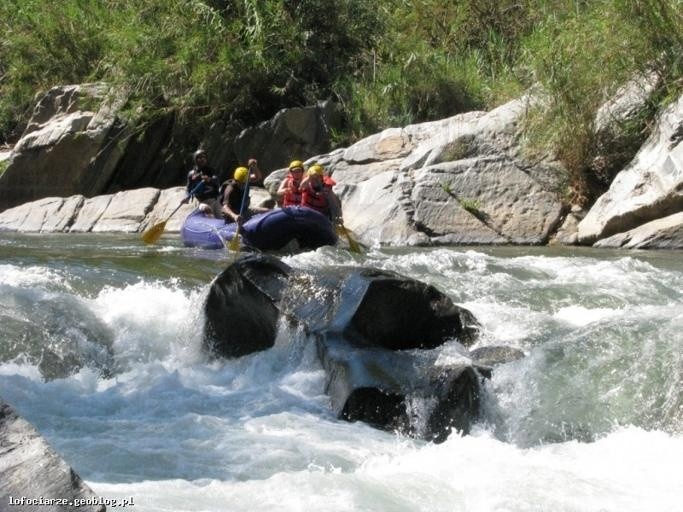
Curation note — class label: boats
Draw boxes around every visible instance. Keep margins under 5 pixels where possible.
[181,202,338,255]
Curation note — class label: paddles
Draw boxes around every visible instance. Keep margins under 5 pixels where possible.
[142,179,206,245]
[229,167,251,252]
[335,211,360,253]
[313,175,355,240]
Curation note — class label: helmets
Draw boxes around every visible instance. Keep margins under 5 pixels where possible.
[194,149,208,162]
[308,166,323,177]
[289,161,304,172]
[234,167,248,184]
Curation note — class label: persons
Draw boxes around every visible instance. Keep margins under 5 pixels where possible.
[277,160,306,206]
[180,149,222,218]
[298,166,342,220]
[218,159,270,224]
